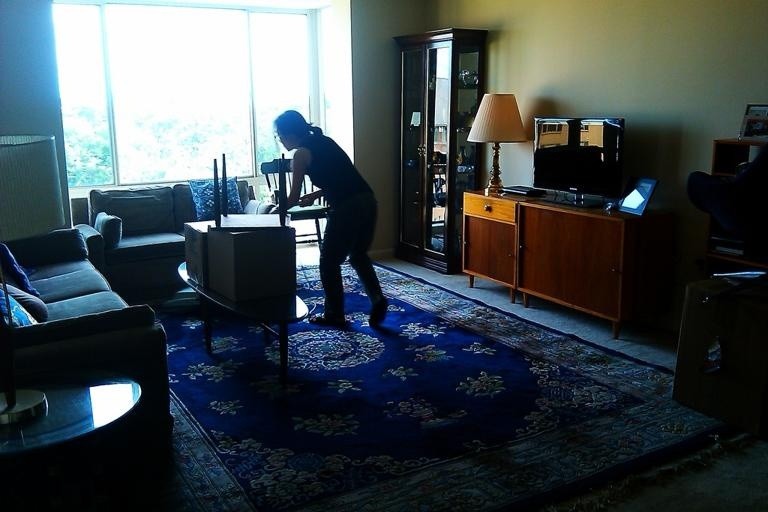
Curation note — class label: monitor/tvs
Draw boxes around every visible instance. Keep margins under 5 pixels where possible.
[533,116,626,209]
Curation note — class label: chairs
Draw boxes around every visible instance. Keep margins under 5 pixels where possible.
[258,159,329,250]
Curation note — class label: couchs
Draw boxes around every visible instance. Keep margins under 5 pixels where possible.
[74,176,272,298]
[0,227,176,458]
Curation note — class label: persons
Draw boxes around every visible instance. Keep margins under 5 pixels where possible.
[269,106,390,329]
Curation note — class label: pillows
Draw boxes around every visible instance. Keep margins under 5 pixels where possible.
[190,176,244,220]
[0,283,48,323]
[0,289,40,329]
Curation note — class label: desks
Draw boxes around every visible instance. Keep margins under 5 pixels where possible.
[0,369,144,512]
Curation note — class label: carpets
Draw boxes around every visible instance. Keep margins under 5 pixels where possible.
[134,259,767,511]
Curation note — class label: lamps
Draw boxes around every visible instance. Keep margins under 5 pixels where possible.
[0,135,67,424]
[467,92,529,194]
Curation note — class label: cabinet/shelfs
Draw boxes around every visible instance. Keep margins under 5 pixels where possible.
[703,135,767,272]
[462,189,540,294]
[395,28,487,273]
[519,203,672,345]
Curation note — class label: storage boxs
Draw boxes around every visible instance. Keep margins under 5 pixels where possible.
[670,275,767,440]
[183,216,296,305]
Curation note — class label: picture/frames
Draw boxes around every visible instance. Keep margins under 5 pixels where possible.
[739,103,768,140]
[620,178,658,215]
